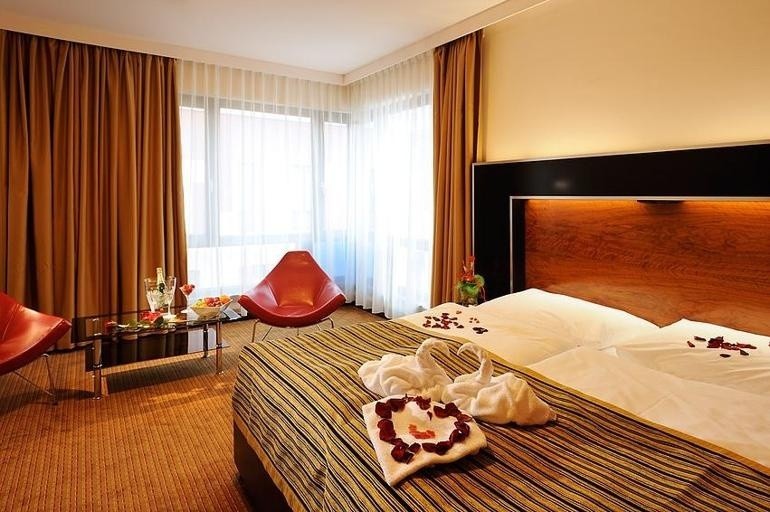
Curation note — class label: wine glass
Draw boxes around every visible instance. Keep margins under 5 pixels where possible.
[179,286,194,314]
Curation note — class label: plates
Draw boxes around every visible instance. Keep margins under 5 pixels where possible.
[190,299,233,319]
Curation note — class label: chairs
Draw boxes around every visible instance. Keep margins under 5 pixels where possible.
[239,249,347,344]
[0,291,73,405]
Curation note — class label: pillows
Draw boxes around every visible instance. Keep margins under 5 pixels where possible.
[476,288,767,397]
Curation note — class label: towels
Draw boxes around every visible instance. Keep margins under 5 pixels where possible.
[357,336,559,488]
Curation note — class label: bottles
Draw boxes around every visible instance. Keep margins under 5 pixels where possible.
[156,267,167,294]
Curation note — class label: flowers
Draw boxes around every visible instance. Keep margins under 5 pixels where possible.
[455,254,486,307]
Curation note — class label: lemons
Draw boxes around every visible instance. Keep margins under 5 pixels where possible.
[194,298,207,308]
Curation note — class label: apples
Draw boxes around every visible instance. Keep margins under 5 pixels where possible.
[203,297,221,307]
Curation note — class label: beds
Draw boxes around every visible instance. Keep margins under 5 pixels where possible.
[230,283,768,512]
